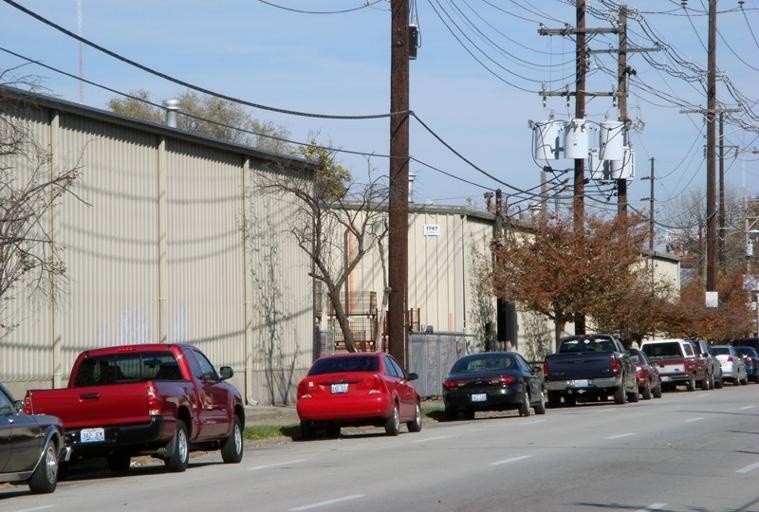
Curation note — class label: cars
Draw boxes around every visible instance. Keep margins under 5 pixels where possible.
[0,382,65,494]
[296,352,422,439]
[543,333,758,408]
[442,351,546,420]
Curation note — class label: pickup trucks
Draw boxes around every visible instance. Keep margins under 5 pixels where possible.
[22,343,245,475]
